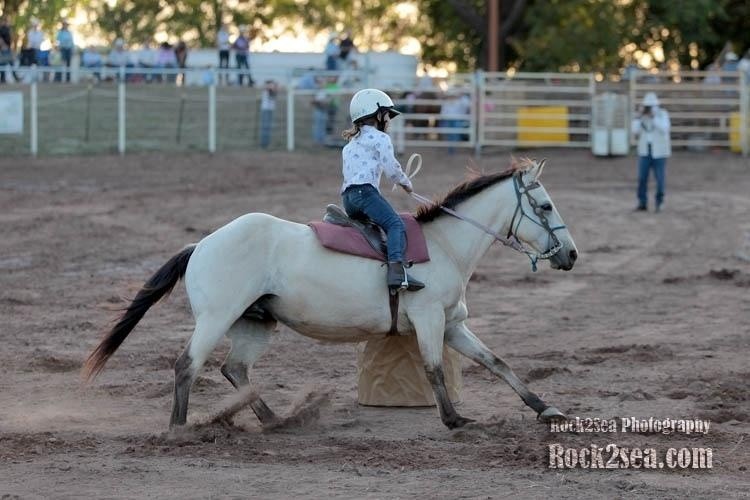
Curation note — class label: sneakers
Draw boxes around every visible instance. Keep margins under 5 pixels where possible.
[632,206,664,214]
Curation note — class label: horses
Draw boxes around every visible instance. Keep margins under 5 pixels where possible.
[77,153,579,430]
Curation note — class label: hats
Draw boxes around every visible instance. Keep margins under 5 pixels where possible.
[641,93,659,106]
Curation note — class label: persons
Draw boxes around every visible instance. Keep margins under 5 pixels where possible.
[175,33,187,86]
[338,32,355,69]
[1,15,75,82]
[258,79,280,150]
[703,50,750,85]
[340,88,427,293]
[231,26,255,85]
[215,22,230,68]
[630,93,672,214]
[324,31,342,69]
[312,72,495,157]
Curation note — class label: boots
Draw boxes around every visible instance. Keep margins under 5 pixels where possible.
[387,262,425,292]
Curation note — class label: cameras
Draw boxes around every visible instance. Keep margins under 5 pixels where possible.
[643,106,651,114]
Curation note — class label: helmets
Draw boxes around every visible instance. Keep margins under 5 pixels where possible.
[350,88,400,124]
